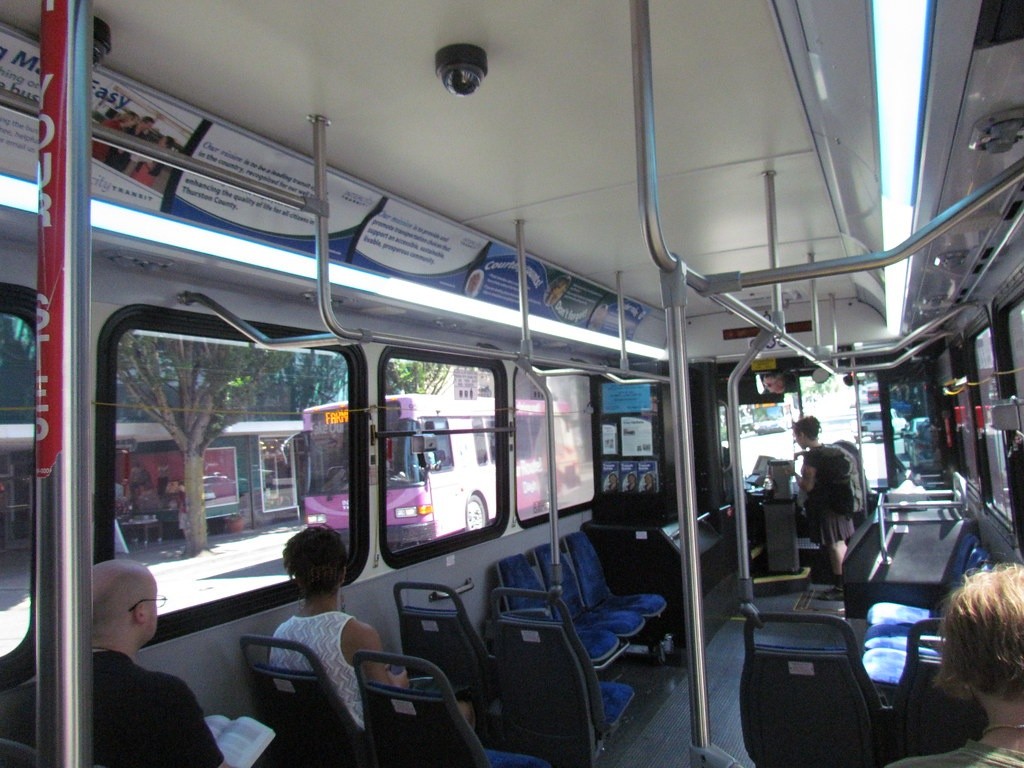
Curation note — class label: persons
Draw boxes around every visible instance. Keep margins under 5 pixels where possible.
[114,480,130,554]
[885,563,1024,768]
[177,480,187,537]
[91,558,228,768]
[643,473,655,491]
[791,417,856,602]
[761,374,796,394]
[123,455,169,508]
[625,473,636,492]
[270,527,476,736]
[605,473,619,492]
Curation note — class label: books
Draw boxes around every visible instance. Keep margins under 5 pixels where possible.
[202,715,276,768]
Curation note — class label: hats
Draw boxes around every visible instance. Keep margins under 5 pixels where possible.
[179,485,185,492]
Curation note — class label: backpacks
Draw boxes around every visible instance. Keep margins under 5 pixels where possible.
[815,449,855,517]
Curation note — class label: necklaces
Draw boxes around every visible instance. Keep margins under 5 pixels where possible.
[983,723,1024,734]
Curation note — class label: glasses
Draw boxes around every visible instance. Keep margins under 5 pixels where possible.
[128,596,166,611]
[762,380,778,388]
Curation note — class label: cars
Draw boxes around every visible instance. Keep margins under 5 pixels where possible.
[861,408,941,475]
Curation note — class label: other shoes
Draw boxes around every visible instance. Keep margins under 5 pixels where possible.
[818,584,846,601]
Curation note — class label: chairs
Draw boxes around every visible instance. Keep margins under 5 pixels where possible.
[1,531,991,767]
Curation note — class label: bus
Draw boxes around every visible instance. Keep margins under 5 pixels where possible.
[747,394,796,435]
[302,393,586,544]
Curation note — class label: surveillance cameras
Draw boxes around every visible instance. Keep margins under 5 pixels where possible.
[435,43,488,97]
[93,18,111,69]
